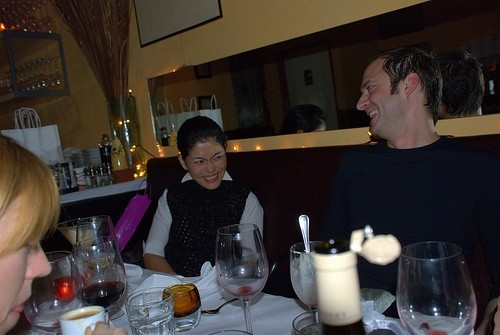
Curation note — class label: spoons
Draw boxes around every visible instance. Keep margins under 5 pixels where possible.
[201,297,241,315]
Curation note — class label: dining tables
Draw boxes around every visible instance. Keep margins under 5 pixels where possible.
[5,262,404,335]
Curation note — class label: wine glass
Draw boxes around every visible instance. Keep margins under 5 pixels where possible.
[0,0,57,33]
[70,236,128,312]
[289,241,336,335]
[215,224,269,335]
[0,54,62,96]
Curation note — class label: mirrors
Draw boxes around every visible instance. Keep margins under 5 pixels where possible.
[146,0,500,147]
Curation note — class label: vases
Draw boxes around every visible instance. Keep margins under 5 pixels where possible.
[106,94,141,178]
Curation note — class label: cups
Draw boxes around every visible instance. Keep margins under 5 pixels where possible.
[59,305,109,335]
[56,216,105,245]
[292,310,322,335]
[125,287,175,335]
[162,283,202,333]
[396,240,477,335]
[207,329,252,335]
[23,250,84,335]
[76,217,119,249]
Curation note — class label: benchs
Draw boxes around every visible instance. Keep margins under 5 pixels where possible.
[144,113,500,300]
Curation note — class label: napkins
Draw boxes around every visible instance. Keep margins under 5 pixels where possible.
[139,262,222,302]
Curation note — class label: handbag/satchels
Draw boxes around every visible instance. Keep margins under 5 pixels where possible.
[0,107,65,168]
[103,179,151,256]
[156,96,224,138]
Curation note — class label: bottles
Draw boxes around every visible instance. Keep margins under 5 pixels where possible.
[311,238,367,335]
[47,129,147,195]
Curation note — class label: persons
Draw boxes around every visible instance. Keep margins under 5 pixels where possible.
[425,47,485,119]
[320,45,500,320]
[0,131,128,335]
[143,115,263,278]
[279,104,327,135]
